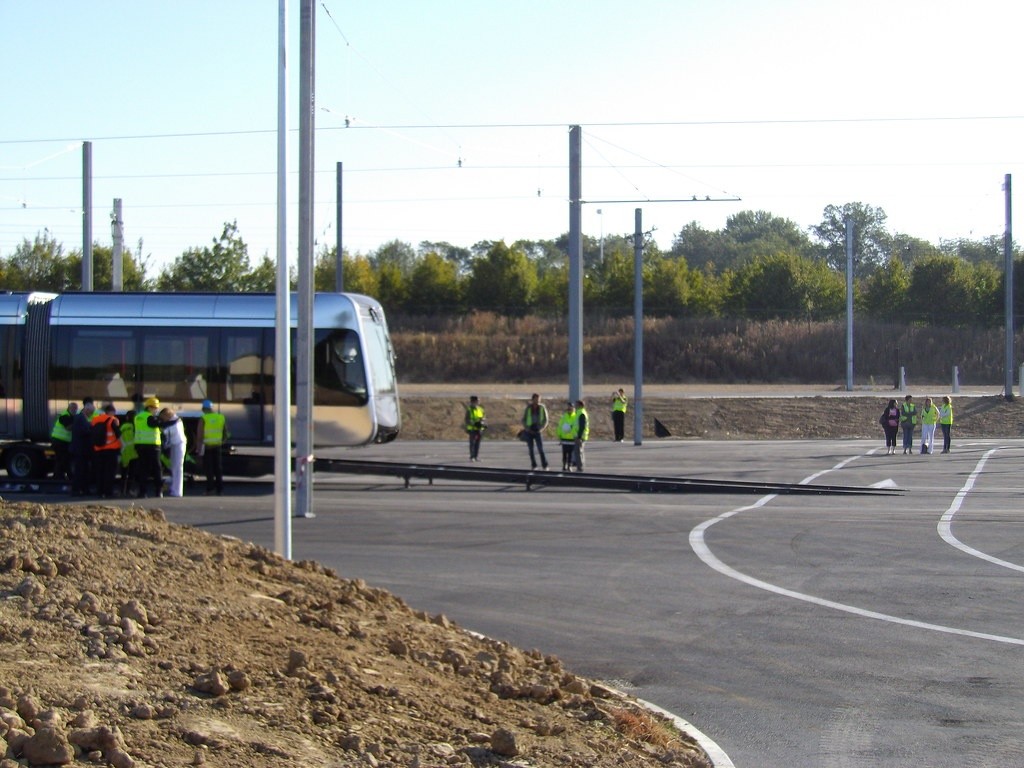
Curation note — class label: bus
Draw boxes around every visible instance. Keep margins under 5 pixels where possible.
[0,288,402,480]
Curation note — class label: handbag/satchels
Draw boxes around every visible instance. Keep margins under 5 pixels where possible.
[517,430,530,441]
[880,414,887,425]
[89,416,113,447]
[922,444,928,453]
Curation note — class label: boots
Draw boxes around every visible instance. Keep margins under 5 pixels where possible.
[891,446,896,454]
[887,447,892,455]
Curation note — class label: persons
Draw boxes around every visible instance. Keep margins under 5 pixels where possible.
[522,393,548,470]
[134,397,180,497]
[465,396,487,462]
[900,395,918,454]
[557,402,576,472]
[572,400,589,471]
[159,408,186,497]
[939,397,952,453]
[196,400,228,496]
[51,398,123,500]
[881,399,900,455]
[611,388,627,442]
[920,398,939,455]
[121,411,139,496]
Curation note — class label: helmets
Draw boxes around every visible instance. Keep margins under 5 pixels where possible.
[144,398,160,409]
[202,399,212,409]
